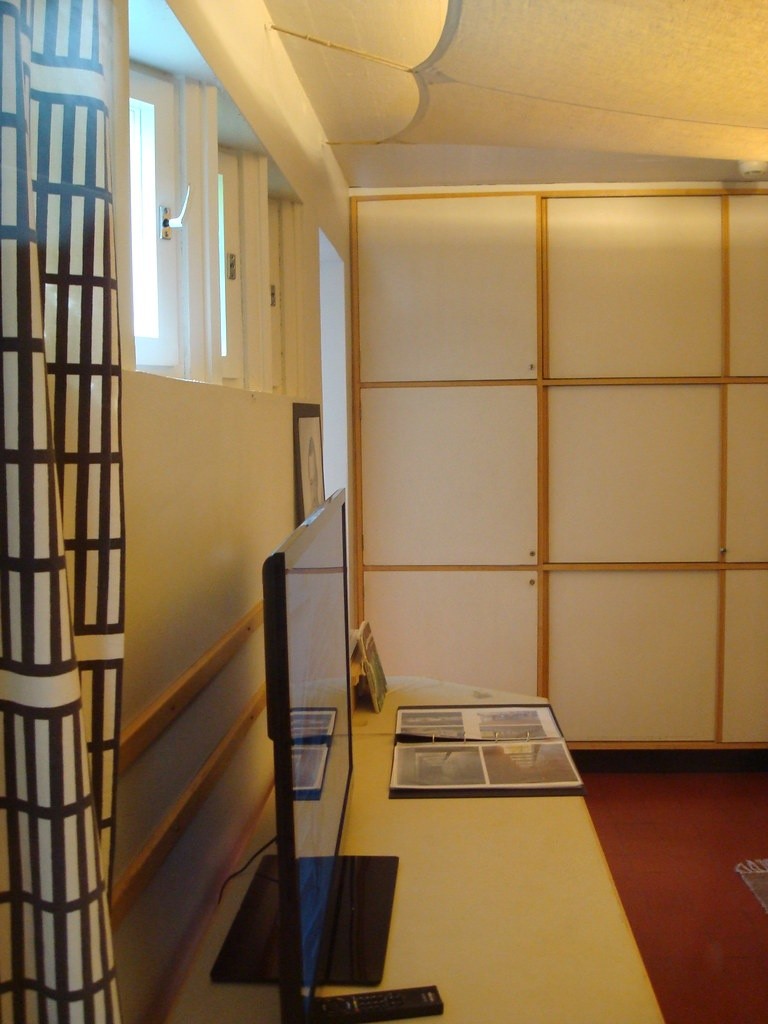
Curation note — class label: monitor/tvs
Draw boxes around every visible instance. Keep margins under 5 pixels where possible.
[203,488,399,1024]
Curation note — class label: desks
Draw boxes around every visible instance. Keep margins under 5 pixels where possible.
[164,675,665,1024]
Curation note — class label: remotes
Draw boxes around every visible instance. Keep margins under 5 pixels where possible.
[314,986,444,1024]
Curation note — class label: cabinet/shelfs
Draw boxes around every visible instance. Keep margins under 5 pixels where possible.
[349,186,768,750]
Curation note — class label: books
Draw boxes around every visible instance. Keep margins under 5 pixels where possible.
[388,704,587,797]
